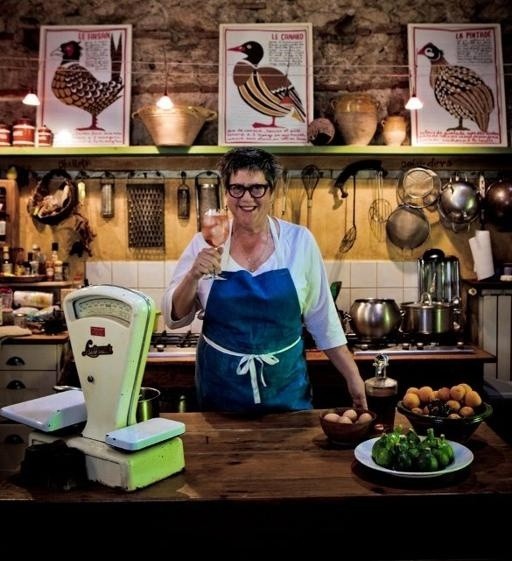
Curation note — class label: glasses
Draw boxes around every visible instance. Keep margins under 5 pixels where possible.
[225,181,271,198]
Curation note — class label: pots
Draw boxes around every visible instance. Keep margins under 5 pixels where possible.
[386,167,512,261]
[349,298,402,340]
[401,291,452,335]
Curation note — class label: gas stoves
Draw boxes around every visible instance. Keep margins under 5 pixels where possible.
[344,331,477,355]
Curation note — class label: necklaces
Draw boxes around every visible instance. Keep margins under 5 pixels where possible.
[233,224,268,273]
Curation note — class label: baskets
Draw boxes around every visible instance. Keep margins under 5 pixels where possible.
[29,168,78,225]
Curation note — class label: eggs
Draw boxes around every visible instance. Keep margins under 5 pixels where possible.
[324,409,371,424]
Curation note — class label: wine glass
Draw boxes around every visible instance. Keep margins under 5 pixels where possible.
[202,213,230,281]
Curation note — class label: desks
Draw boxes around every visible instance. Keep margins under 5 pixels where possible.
[0,411,511,504]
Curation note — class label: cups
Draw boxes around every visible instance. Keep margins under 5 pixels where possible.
[0,117,53,147]
[136,386,160,422]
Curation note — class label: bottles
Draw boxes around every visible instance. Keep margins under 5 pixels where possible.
[0,242,69,282]
[1,307,27,330]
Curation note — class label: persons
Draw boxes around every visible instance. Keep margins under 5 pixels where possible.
[160,146,370,412]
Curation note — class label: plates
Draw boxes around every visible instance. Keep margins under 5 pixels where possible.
[354,432,473,479]
[319,408,377,446]
[397,400,492,444]
